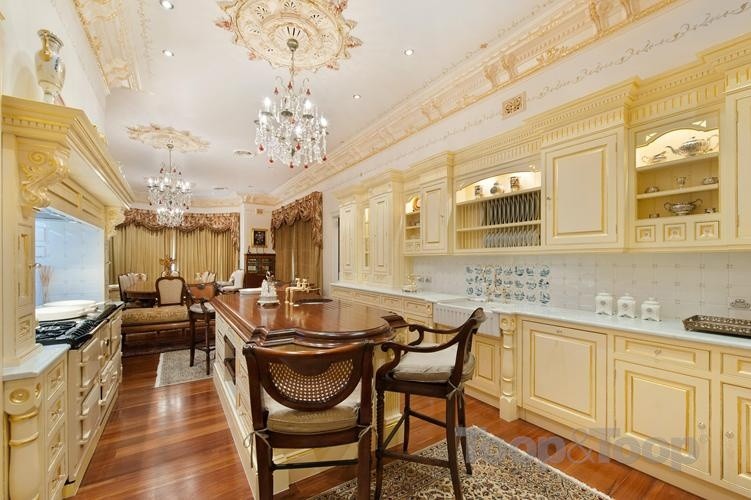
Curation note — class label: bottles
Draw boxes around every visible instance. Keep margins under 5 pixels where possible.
[596,289,614,316]
[617,293,636,319]
[641,297,661,321]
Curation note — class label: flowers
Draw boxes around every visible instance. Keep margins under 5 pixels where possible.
[158,253,177,268]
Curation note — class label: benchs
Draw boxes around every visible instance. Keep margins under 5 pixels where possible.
[121,306,216,347]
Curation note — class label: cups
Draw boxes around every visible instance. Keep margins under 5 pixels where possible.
[705,208,715,213]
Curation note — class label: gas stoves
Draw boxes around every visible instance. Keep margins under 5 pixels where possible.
[35,301,125,350]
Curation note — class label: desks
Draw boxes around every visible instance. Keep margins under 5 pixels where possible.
[123,280,158,304]
[184,277,216,289]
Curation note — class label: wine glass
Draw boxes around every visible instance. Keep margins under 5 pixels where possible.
[676,176,687,189]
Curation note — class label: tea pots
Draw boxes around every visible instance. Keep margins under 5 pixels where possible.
[665,134,719,157]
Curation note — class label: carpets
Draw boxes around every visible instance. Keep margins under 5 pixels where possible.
[152,348,216,388]
[302,423,620,500]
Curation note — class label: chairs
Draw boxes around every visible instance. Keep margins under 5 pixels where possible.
[217,269,245,295]
[118,271,147,302]
[162,270,180,277]
[194,271,216,283]
[155,276,186,306]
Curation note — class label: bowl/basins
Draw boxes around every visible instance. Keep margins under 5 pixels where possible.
[703,177,719,185]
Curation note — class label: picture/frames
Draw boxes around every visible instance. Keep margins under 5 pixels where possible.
[251,226,268,249]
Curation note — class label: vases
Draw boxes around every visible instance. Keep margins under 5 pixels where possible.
[35,26,66,104]
[161,268,175,278]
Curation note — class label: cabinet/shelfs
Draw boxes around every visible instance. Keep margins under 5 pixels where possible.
[628,109,725,243]
[0,305,124,500]
[400,169,452,255]
[329,284,751,500]
[244,252,277,289]
[451,154,545,253]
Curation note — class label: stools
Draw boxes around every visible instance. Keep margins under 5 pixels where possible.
[186,283,224,376]
[241,340,375,500]
[374,304,488,500]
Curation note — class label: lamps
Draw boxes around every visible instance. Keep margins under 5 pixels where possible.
[145,139,195,230]
[252,21,332,171]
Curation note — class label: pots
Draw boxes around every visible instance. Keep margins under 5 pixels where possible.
[35,299,111,322]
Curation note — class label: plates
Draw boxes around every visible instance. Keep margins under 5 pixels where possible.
[238,288,262,294]
